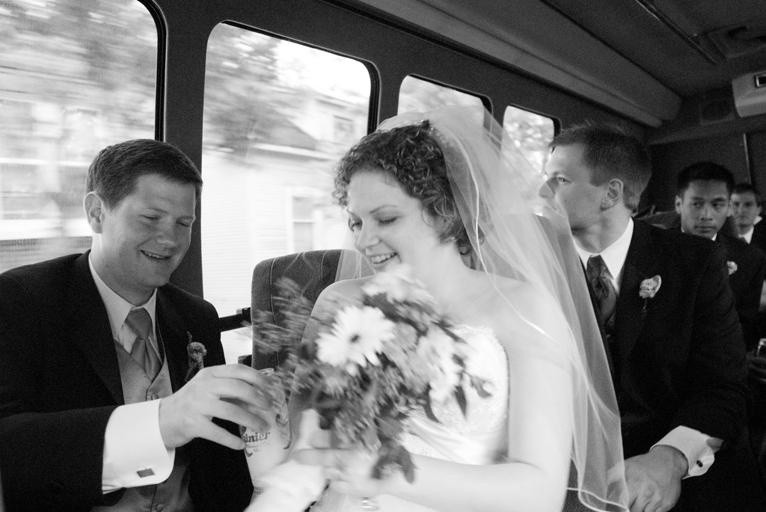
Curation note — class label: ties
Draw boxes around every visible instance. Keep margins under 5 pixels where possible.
[125,308,162,382]
[585,255,620,344]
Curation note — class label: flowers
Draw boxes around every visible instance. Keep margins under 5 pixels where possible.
[240,261,495,486]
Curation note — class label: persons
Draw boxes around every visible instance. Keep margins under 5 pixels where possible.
[0,138,276,512]
[239,103,635,512]
[529,119,752,512]
[727,181,765,243]
[661,158,766,353]
